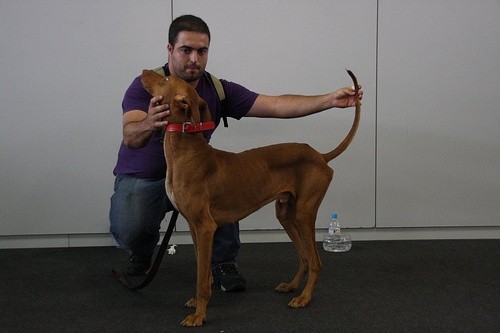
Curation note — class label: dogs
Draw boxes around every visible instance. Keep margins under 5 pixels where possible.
[140,68,361,328]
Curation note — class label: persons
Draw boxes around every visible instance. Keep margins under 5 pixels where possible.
[109,14,364,292]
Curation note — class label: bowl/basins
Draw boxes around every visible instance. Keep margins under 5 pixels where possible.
[323,235,352,252]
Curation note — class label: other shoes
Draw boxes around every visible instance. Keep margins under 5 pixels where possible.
[212,263,246,292]
[127,251,154,276]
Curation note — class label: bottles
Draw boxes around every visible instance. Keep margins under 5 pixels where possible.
[329,213,341,236]
